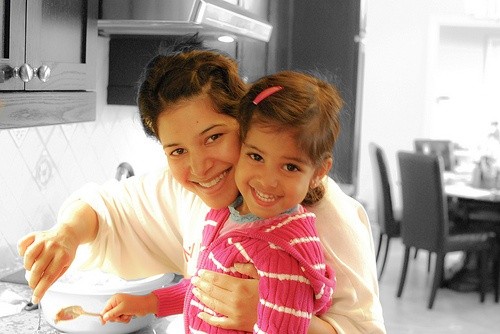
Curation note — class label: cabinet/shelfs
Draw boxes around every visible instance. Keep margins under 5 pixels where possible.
[0,0,99,130]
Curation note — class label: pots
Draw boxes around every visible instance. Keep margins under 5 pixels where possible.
[25,250,175,333]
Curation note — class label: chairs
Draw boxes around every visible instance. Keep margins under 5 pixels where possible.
[374,146,401,281]
[398,151,491,309]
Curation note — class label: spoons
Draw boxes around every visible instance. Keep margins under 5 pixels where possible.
[57,305,102,325]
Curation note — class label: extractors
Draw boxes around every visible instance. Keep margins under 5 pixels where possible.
[97,0,274,44]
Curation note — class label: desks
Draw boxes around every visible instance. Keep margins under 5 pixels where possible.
[440,186,500,292]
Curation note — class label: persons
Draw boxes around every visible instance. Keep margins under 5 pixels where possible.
[99,70,343,334]
[17,32,387,334]
